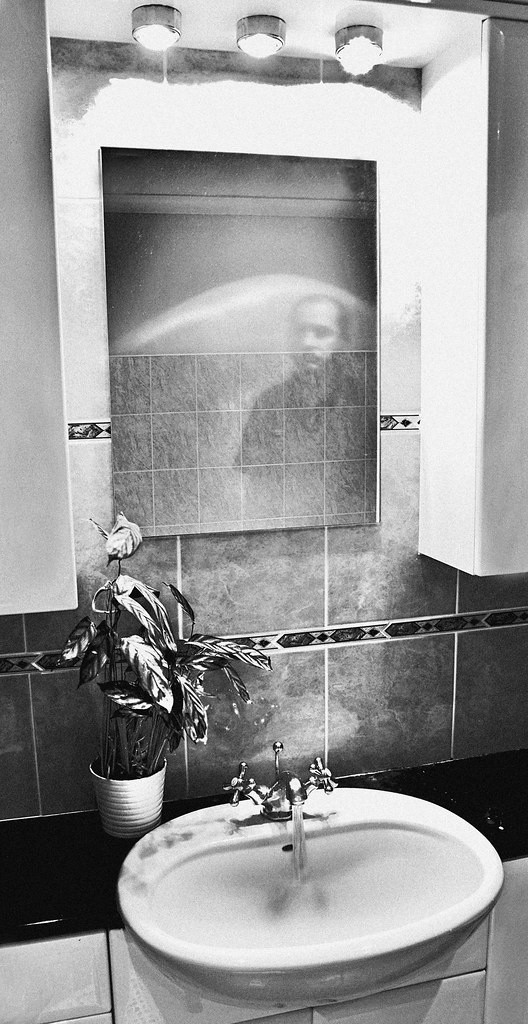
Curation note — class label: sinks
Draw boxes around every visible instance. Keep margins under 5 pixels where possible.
[114,787,510,1012]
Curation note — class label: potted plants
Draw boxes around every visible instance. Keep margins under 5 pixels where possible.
[50,509,274,839]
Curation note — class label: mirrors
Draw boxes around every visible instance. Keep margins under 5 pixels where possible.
[98,146,377,540]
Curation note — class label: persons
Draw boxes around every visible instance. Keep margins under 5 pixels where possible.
[233,294,376,524]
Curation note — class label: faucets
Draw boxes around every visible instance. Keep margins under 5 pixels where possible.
[263,739,309,823]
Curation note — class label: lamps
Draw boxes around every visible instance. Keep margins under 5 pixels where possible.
[236,14,286,59]
[335,24,384,76]
[131,4,183,51]
